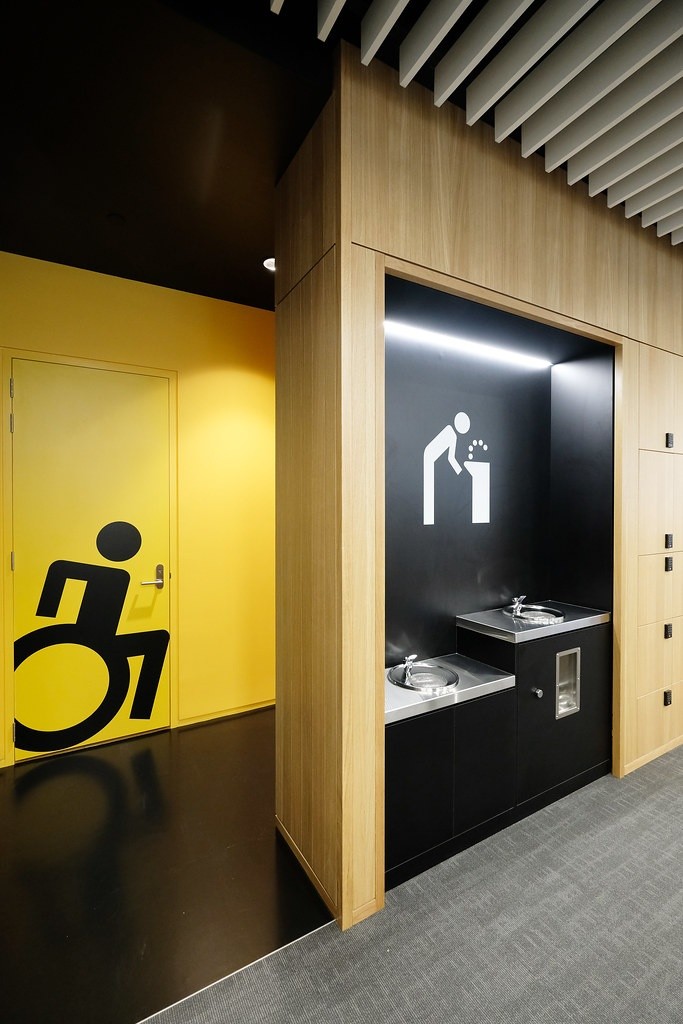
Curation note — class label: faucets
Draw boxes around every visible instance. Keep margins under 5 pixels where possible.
[511,595,527,615]
[401,654,418,687]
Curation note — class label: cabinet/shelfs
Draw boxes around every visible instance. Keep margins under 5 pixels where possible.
[383,621,615,872]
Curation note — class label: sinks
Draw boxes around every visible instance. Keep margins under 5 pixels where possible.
[388,662,460,692]
[502,604,565,622]
